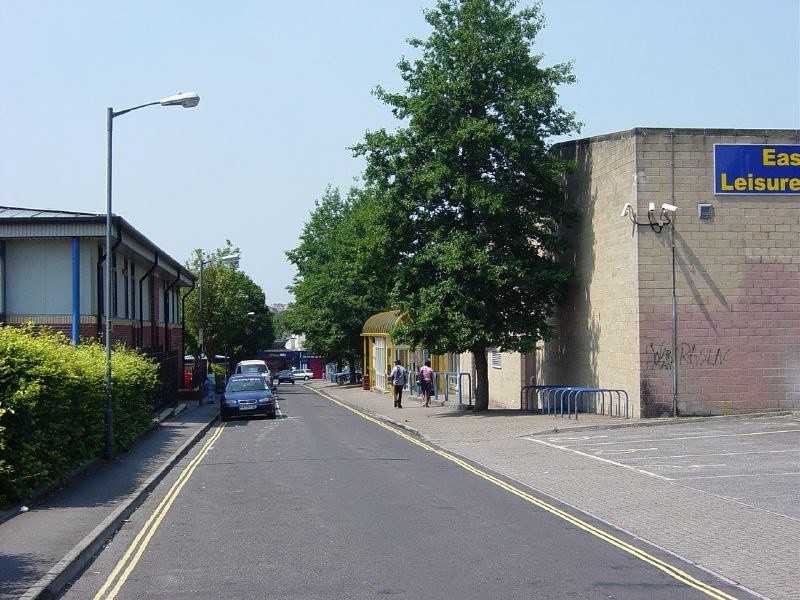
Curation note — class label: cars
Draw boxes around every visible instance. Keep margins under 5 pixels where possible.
[276,369,296,385]
[215,371,278,422]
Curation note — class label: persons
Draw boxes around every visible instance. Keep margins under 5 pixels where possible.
[391,360,407,408]
[419,360,434,408]
[204,366,217,404]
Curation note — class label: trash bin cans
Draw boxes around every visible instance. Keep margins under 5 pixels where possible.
[363,375,370,390]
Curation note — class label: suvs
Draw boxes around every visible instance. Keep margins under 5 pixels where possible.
[292,368,315,381]
[226,360,276,391]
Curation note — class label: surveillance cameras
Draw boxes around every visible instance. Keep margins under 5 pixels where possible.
[662,203,679,212]
[649,202,655,212]
[622,203,630,218]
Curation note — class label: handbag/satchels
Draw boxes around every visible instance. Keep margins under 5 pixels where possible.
[394,365,402,384]
[417,366,424,386]
[430,390,435,396]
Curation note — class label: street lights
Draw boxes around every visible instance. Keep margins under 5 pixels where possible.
[198,253,239,406]
[104,91,200,462]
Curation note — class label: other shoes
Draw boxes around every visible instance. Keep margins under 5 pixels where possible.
[425,405,429,407]
[398,405,402,408]
[208,400,216,404]
[422,403,425,407]
[394,404,397,407]
